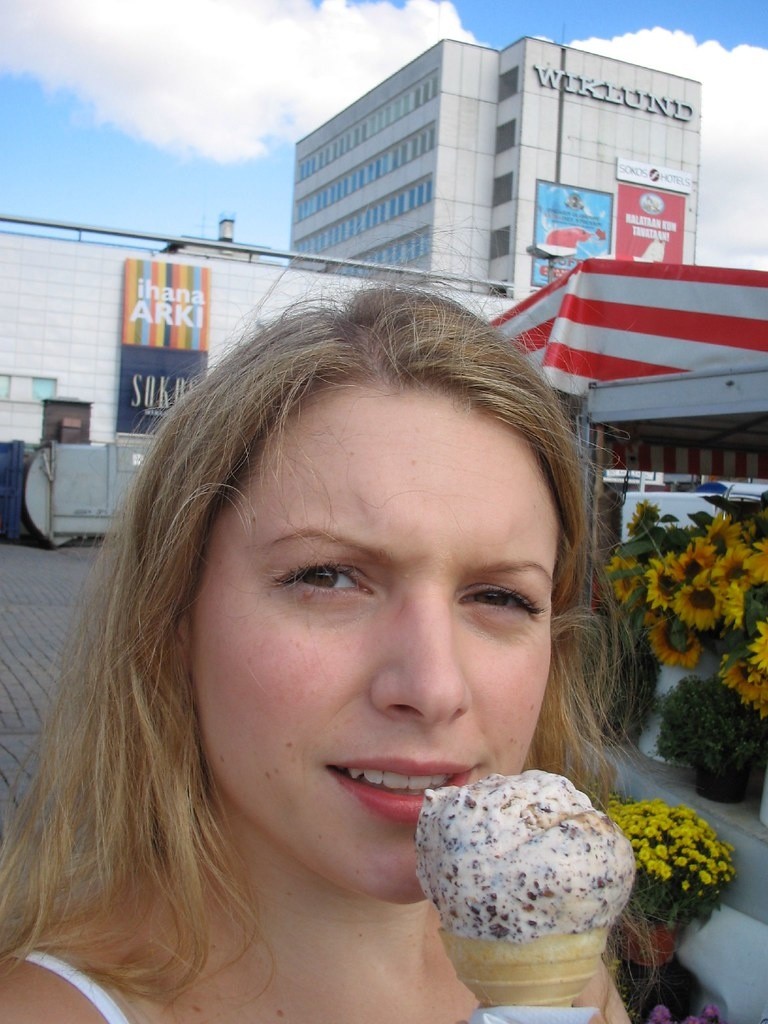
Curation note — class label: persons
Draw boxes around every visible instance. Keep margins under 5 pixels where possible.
[0,289,637,1024]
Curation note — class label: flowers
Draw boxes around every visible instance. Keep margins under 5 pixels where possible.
[602,485,768,721]
[590,779,738,1024]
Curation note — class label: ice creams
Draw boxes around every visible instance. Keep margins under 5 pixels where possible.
[416,769,636,1010]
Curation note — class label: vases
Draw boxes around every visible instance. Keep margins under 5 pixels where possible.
[621,916,678,967]
[637,646,722,771]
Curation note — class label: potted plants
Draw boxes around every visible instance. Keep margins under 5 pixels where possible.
[650,675,768,803]
